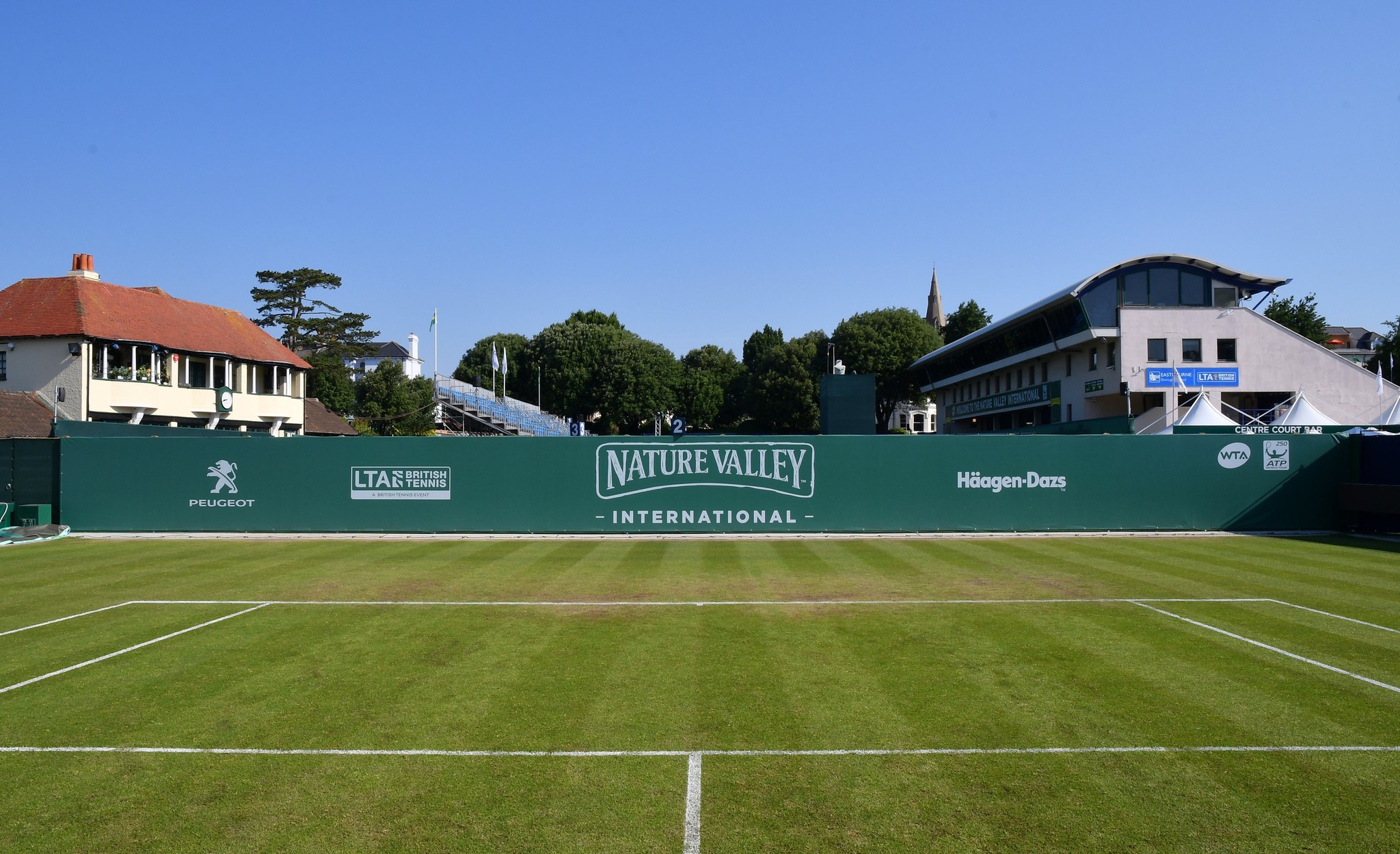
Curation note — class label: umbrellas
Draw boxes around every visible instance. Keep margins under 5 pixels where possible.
[1326,339,1344,350]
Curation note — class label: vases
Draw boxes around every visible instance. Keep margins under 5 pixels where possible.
[162,379,168,385]
[137,376,147,380]
[115,375,126,380]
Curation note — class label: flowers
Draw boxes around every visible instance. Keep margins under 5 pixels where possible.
[109,363,170,380]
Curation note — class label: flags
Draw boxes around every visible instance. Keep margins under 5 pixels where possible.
[1391,354,1393,368]
[502,349,507,375]
[1173,366,1188,394]
[492,344,499,371]
[1376,365,1383,395]
[1353,353,1361,363]
[537,370,540,384]
[430,312,435,332]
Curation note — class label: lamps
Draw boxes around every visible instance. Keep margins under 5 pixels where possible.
[989,372,994,377]
[68,343,82,356]
[149,345,157,354]
[1035,357,1040,362]
[954,383,958,388]
[111,342,121,350]
[160,349,169,358]
[8,343,14,351]
[1099,337,1107,344]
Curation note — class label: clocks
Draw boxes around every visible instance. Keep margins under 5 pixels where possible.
[221,391,232,409]
